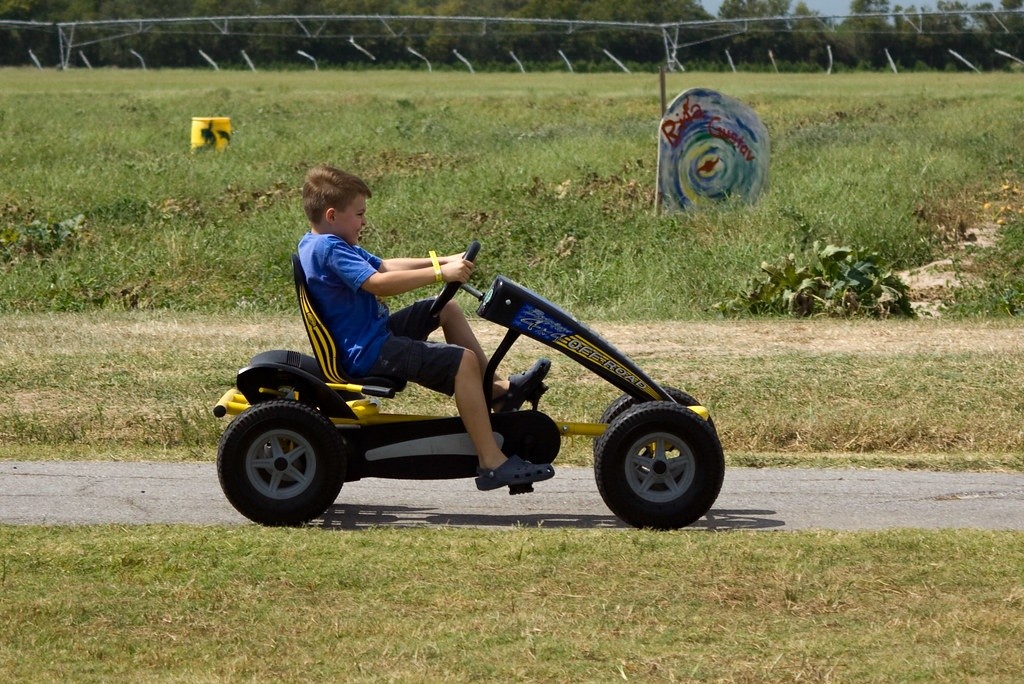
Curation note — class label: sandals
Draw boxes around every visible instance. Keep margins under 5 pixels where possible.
[492,357,554,413]
[474,455,555,492]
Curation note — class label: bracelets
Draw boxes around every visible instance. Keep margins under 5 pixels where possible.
[429,251,442,283]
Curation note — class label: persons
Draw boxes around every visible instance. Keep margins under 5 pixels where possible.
[296,164,555,491]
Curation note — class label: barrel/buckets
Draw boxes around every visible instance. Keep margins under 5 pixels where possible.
[191,116,232,151]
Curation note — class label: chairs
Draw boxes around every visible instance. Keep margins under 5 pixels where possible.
[291,255,406,398]
